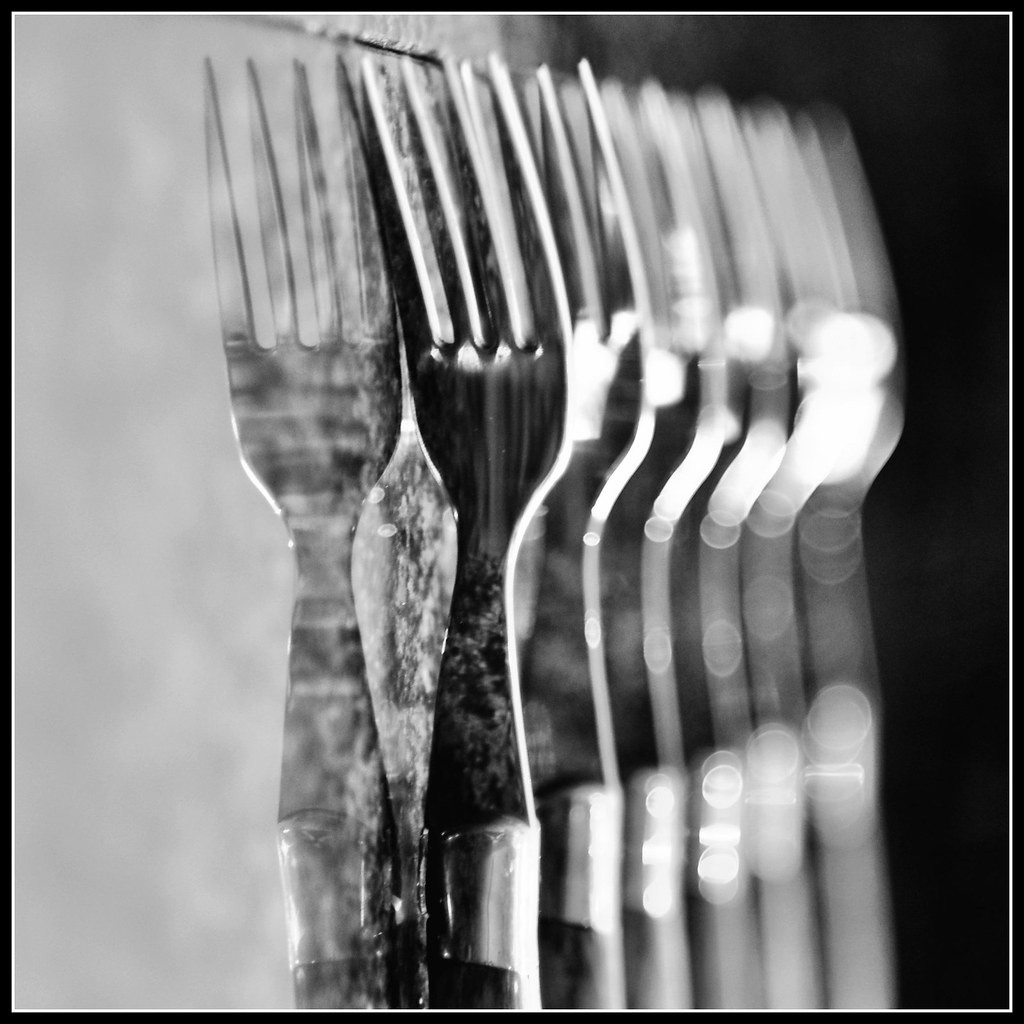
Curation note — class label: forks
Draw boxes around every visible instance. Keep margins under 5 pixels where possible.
[191,49,905,1010]
